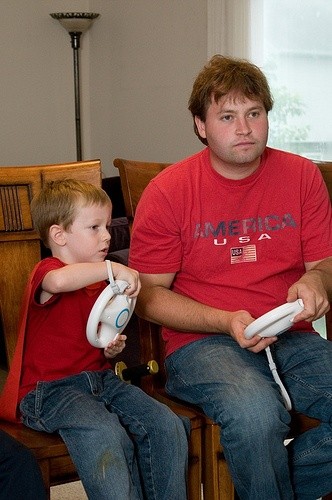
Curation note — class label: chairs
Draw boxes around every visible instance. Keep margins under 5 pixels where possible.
[113,157,331,496]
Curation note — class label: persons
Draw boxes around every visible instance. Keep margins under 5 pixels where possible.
[129,54,331,499]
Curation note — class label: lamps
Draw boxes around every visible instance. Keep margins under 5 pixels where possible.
[48,10,101,162]
